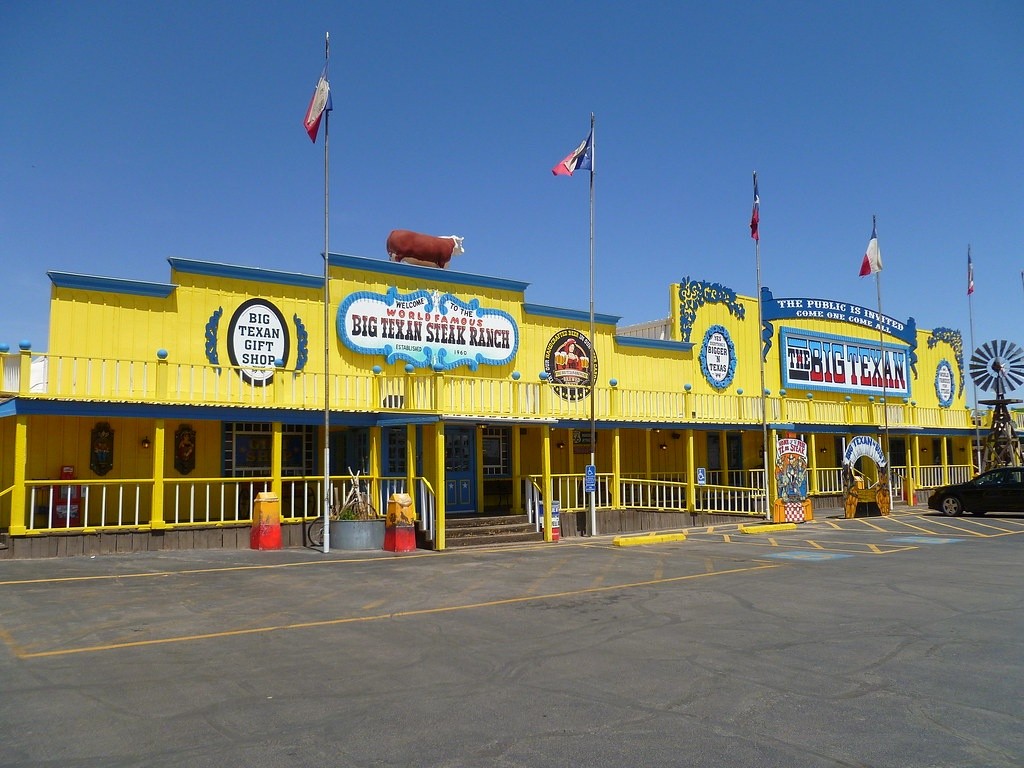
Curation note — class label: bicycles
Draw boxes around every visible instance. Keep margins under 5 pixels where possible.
[308,465,380,548]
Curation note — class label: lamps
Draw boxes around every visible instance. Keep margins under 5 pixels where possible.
[820,445,828,454]
[959,446,965,452]
[557,440,565,449]
[653,428,662,434]
[475,423,490,429]
[660,442,667,450]
[921,448,927,453]
[142,436,151,449]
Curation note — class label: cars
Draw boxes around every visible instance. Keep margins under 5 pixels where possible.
[928,466,1024,518]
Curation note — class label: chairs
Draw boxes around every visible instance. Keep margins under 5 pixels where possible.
[28,477,54,528]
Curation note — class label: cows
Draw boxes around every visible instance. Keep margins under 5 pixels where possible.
[386,229,466,270]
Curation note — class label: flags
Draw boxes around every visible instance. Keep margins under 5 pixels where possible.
[967,251,974,294]
[750,183,759,239]
[552,131,592,177]
[859,227,883,276]
[303,56,333,144]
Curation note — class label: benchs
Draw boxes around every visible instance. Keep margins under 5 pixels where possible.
[483,480,525,508]
